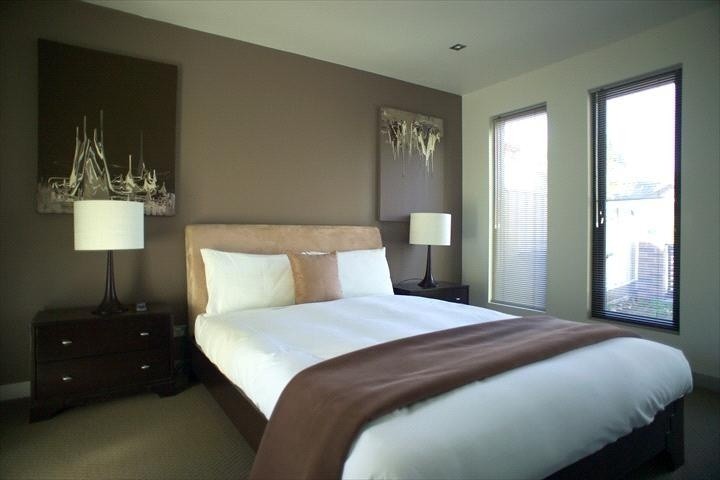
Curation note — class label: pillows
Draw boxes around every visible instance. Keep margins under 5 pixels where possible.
[284,249,344,304]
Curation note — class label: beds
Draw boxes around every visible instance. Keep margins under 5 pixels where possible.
[184,222,694,480]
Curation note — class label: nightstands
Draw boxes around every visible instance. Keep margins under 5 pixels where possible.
[396,280,470,305]
[28,302,180,423]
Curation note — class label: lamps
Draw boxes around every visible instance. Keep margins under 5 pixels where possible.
[409,212,452,286]
[72,199,145,315]
